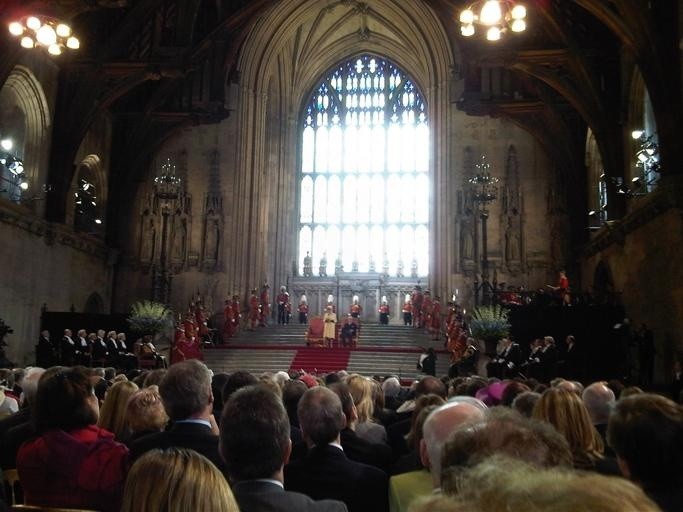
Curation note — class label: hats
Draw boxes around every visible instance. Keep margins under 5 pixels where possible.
[476,389,493,407]
[299,375,317,388]
[488,381,510,400]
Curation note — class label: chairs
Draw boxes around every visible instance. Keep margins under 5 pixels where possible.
[338,318,360,349]
[88,341,112,368]
[135,343,159,371]
[305,318,324,347]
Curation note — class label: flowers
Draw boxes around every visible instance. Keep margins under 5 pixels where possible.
[467,305,513,340]
[126,299,171,332]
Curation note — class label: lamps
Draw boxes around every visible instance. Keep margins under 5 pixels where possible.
[8,14,81,56]
[73,177,102,225]
[1,139,30,191]
[459,0,527,41]
[631,128,661,188]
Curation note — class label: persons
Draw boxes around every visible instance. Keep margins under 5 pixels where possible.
[351,300,360,318]
[342,316,356,347]
[379,301,390,323]
[40,329,170,368]
[298,302,308,324]
[223,283,291,337]
[325,302,335,314]
[402,266,683,369]
[323,305,338,348]
[1,359,683,512]
[170,293,207,363]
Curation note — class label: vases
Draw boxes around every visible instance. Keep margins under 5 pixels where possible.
[486,342,497,355]
[142,334,152,342]
